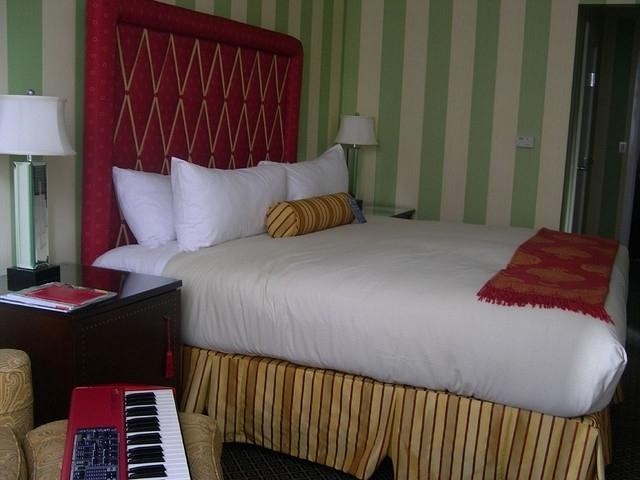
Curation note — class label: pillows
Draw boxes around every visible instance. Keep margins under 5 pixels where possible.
[169,155,291,252]
[257,145,353,202]
[263,192,360,239]
[111,164,176,250]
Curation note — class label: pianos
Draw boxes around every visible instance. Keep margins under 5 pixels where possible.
[60,385,193,476]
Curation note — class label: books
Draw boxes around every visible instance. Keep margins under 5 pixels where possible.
[0,282,117,314]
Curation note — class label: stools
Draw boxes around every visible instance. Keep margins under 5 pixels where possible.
[18,408,227,480]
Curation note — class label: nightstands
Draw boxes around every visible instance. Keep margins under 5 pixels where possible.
[1,260,185,427]
[363,204,418,221]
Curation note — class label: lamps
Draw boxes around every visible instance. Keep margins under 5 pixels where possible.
[0,89,78,293]
[332,112,380,213]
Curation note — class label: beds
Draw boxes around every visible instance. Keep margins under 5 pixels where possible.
[81,0,633,480]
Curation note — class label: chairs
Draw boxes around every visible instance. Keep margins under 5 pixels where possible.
[0,348,40,480]
